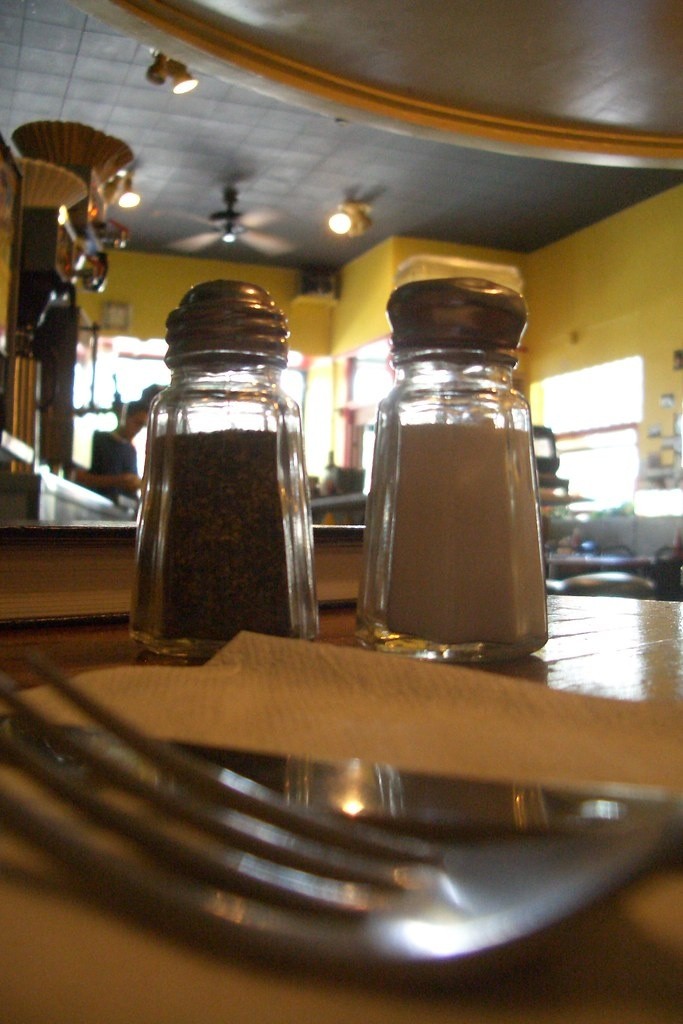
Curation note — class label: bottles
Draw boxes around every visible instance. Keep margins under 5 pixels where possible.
[357,276,549,663]
[125,276,319,667]
[325,451,337,474]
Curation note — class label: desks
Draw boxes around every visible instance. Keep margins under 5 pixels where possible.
[0,593,683,1024]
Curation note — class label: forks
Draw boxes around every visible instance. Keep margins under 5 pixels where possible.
[0,645,683,973]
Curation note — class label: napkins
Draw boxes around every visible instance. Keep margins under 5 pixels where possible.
[0,628,683,792]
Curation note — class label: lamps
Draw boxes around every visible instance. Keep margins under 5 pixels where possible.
[117,176,141,208]
[145,53,198,94]
[329,199,374,238]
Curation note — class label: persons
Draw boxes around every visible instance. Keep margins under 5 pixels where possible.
[75,400,149,502]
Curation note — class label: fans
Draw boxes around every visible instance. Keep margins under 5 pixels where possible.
[155,185,298,257]
